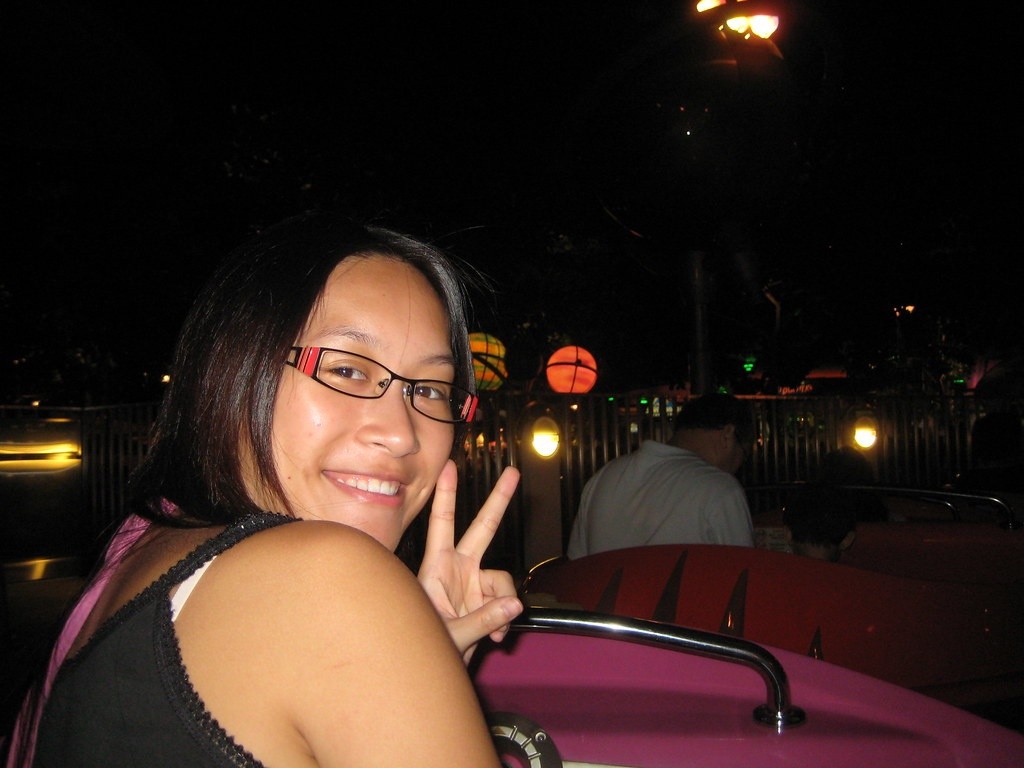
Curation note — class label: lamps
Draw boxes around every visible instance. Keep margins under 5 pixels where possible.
[546,345,600,394]
[466,331,508,394]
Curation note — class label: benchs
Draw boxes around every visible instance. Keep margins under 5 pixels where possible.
[746,510,1023,582]
[545,543,1024,683]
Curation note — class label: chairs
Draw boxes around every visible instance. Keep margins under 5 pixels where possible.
[475,618,1024,768]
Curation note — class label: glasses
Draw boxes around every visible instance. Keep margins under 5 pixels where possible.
[734,433,753,471]
[282,345,479,423]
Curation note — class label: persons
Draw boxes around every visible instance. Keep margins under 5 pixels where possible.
[566,392,757,563]
[783,486,857,562]
[0,218,526,768]
